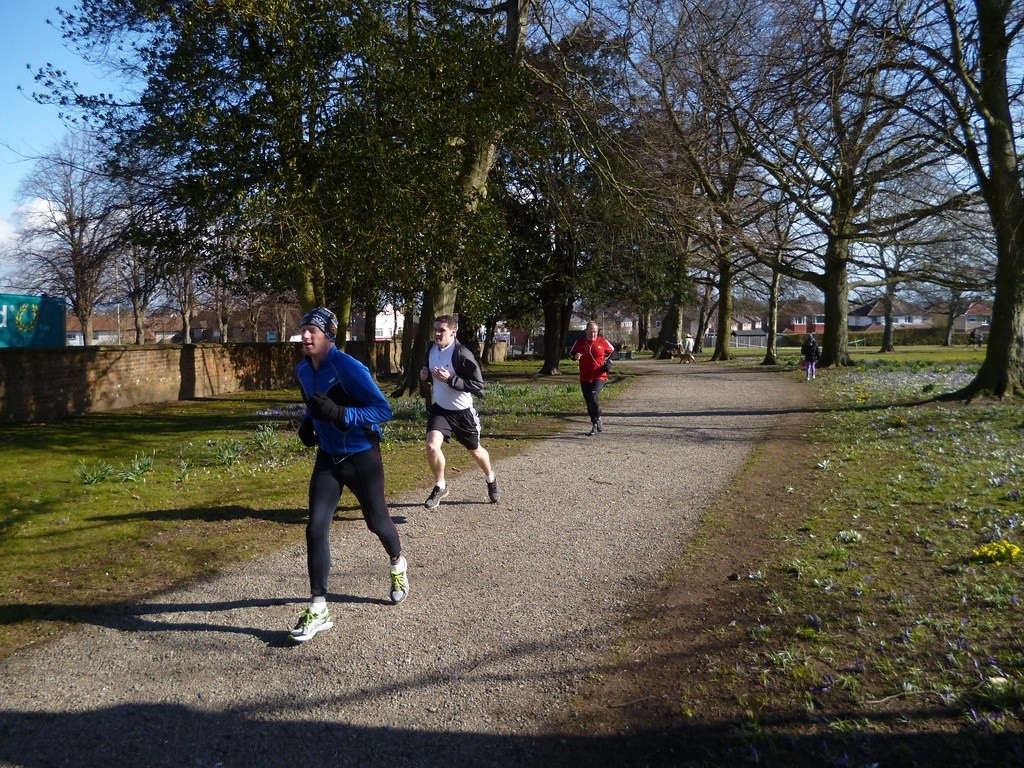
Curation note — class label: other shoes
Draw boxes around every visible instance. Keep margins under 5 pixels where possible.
[812,376,816,379]
[596,417,602,432]
[692,360,696,363]
[589,427,597,436]
[806,377,811,380]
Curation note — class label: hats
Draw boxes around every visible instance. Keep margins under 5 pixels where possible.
[298,307,338,342]
[686,333,692,337]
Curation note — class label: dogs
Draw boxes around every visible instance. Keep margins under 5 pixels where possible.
[678,352,695,364]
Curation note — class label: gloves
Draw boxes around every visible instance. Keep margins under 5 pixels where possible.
[298,417,320,447]
[307,390,346,426]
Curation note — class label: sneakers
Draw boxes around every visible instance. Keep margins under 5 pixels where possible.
[287,607,334,642]
[424,483,449,509]
[485,471,501,503]
[388,555,410,604]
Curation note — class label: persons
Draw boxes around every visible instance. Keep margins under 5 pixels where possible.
[684,334,696,363]
[963,329,984,350]
[569,320,615,436]
[420,315,501,510]
[800,334,822,380]
[288,306,410,643]
